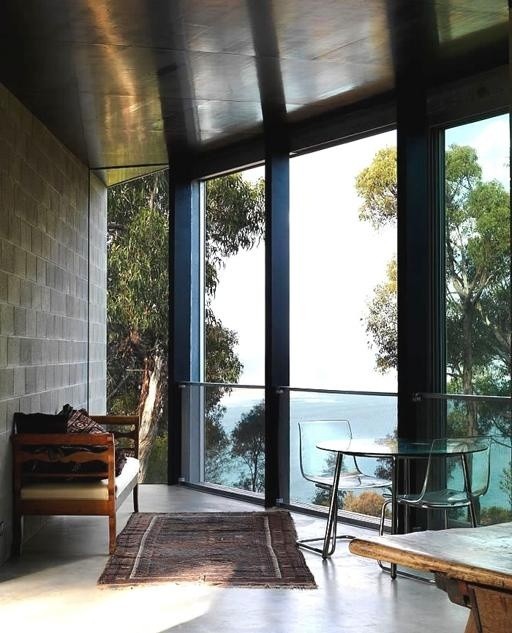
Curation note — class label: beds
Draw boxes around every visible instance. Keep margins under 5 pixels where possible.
[8,414,141,560]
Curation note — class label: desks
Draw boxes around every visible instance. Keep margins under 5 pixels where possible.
[312,437,489,578]
[349,520,511,632]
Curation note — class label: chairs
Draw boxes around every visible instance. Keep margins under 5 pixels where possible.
[295,418,398,561]
[377,435,495,587]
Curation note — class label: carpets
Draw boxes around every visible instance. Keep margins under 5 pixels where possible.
[97,510,318,590]
